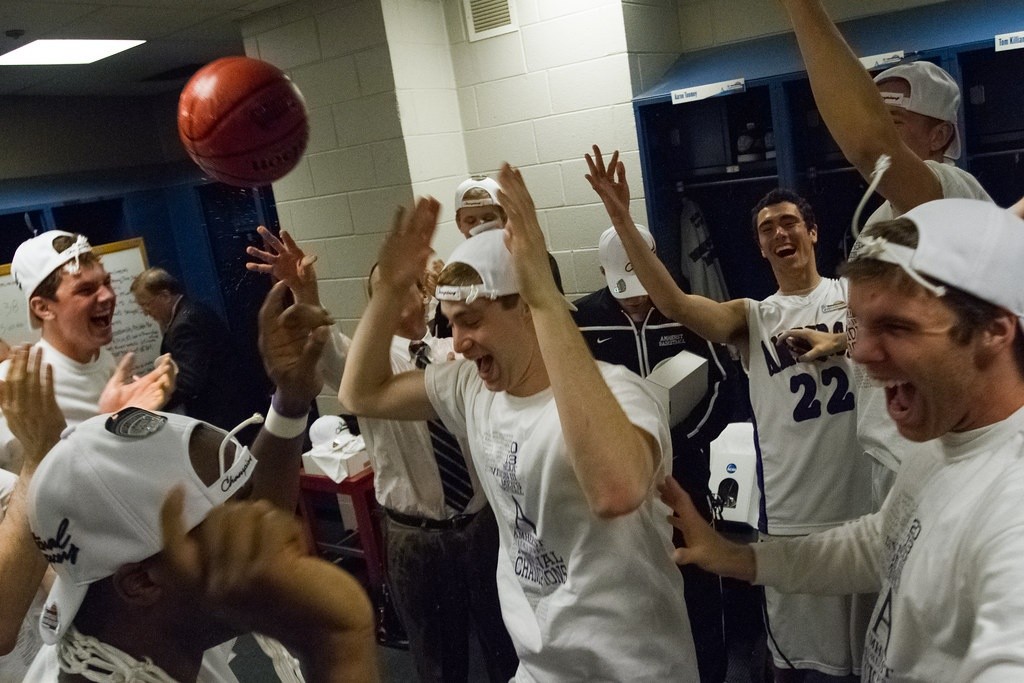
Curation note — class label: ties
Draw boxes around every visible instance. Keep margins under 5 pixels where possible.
[410,340,475,513]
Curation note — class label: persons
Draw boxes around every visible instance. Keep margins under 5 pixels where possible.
[1,0,1023,682]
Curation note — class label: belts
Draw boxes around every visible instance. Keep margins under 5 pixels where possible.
[385,507,480,529]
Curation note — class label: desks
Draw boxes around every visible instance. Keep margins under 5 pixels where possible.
[296,468,396,646]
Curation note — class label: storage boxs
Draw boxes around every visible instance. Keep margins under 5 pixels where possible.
[301,449,372,478]
[645,349,708,428]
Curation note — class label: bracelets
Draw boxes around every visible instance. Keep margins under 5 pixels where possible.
[264,394,311,439]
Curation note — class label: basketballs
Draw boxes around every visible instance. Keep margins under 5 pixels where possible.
[175,54,311,189]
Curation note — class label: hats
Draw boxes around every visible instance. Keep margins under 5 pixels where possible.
[27,404,264,646]
[599,225,657,299]
[433,229,578,312]
[11,230,90,330]
[870,61,962,159]
[309,413,357,453]
[455,175,503,212]
[855,198,1024,332]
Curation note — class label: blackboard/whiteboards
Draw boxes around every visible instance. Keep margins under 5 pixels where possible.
[0,236,163,389]
